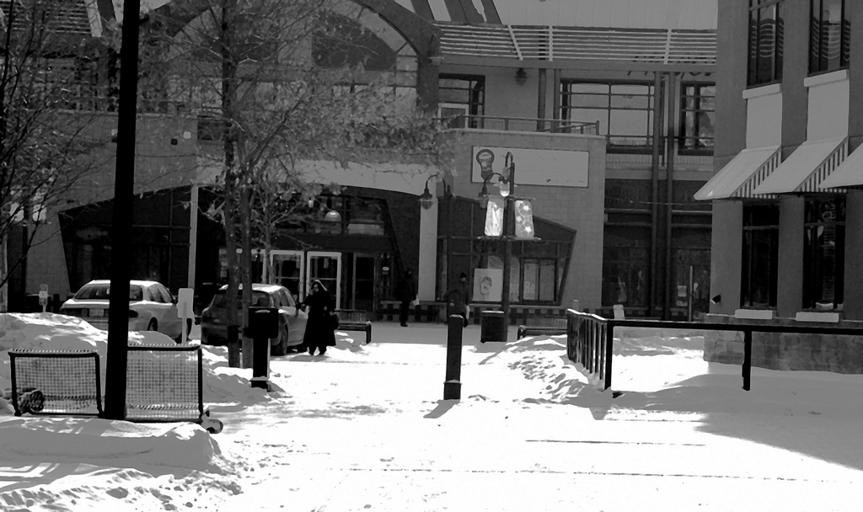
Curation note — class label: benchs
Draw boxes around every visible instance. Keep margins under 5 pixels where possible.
[517,314,567,341]
[335,310,371,344]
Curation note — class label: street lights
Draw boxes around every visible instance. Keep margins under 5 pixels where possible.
[479,145,516,345]
[418,166,453,324]
[275,185,343,311]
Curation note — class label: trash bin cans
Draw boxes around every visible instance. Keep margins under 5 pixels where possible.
[480,310,507,343]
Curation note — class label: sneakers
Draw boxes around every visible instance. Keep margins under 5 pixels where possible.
[309,348,326,356]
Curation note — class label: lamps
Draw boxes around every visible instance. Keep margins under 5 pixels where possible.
[514,68,528,86]
[325,210,341,222]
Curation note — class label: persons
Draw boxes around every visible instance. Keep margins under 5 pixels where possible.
[398,266,416,327]
[372,265,394,321]
[443,278,469,328]
[303,279,336,356]
[456,272,470,316]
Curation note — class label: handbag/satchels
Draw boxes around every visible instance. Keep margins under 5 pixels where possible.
[330,312,339,330]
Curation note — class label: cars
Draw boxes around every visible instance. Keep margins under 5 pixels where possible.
[199,279,309,353]
[61,277,193,345]
